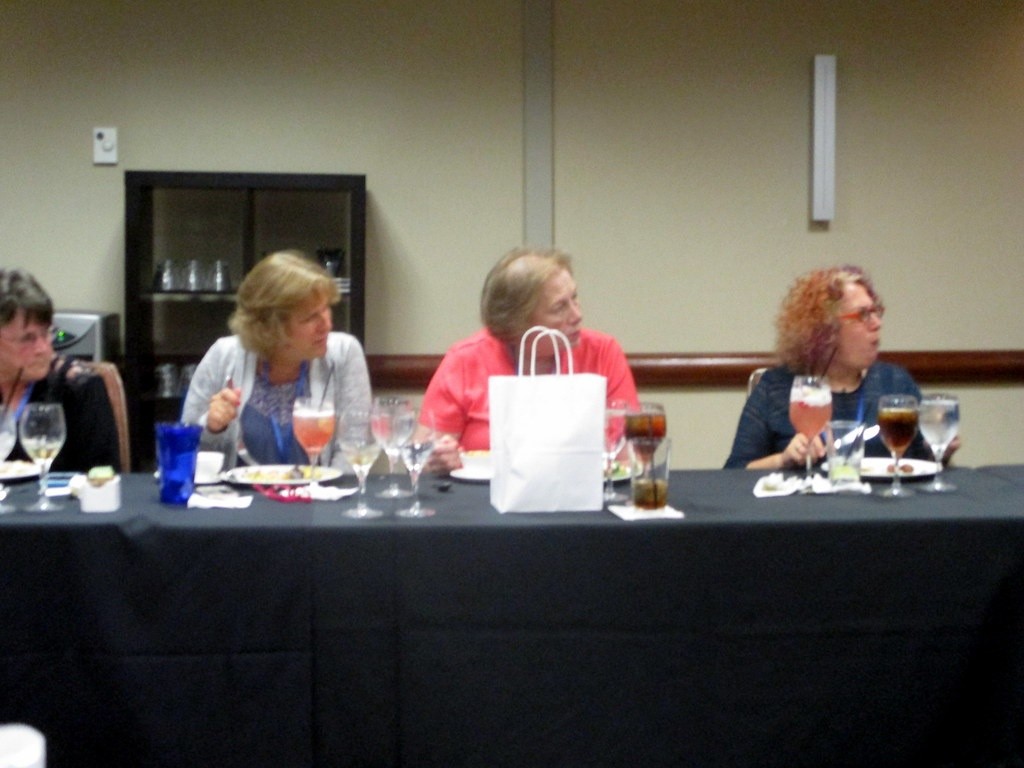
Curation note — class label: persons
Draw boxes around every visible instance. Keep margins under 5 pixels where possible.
[0,265,121,476]
[721,265,961,470]
[180,250,375,479]
[412,248,642,481]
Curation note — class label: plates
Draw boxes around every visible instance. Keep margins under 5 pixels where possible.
[0,462,39,479]
[226,465,342,485]
[820,457,942,478]
[449,469,490,480]
[154,470,225,484]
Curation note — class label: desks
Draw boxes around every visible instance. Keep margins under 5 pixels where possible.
[0,466,1024,768]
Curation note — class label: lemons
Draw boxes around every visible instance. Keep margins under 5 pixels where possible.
[833,466,859,480]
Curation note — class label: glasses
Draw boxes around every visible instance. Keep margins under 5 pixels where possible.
[840,300,886,326]
[0,324,65,349]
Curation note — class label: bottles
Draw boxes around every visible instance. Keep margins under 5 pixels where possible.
[154,422,203,504]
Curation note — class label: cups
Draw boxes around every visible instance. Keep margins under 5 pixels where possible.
[156,259,227,398]
[828,421,863,483]
[631,437,670,509]
[194,451,225,474]
[318,249,342,278]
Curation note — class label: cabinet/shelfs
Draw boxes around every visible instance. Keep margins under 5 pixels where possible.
[123,170,366,470]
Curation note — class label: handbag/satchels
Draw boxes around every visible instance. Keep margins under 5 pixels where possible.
[487,325,606,513]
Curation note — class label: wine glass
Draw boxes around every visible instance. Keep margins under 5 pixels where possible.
[878,395,918,498]
[625,403,667,476]
[338,406,383,519]
[789,375,832,477]
[603,401,627,500]
[373,398,414,498]
[19,403,66,513]
[293,397,336,485]
[394,409,437,518]
[918,398,960,492]
[0,403,17,515]
